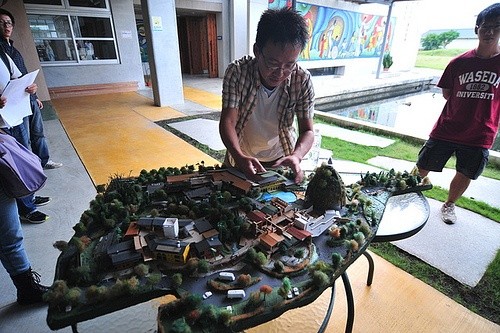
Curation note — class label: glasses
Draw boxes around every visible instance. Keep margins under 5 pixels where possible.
[260,48,299,73]
[0,21,14,27]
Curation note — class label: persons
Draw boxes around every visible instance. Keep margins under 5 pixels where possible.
[0,52,52,307]
[410,3,500,225]
[219,6,314,185]
[0,10,63,169]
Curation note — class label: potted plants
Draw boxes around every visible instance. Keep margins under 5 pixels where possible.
[382,53,393,71]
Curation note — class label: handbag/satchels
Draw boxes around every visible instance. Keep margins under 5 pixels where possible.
[0,132,48,199]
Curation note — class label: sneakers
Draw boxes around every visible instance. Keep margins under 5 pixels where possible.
[19,209,47,224]
[45,160,62,168]
[440,203,457,223]
[33,195,52,206]
[16,284,52,304]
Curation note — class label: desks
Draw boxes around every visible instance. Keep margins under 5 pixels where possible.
[72,171,429,333]
